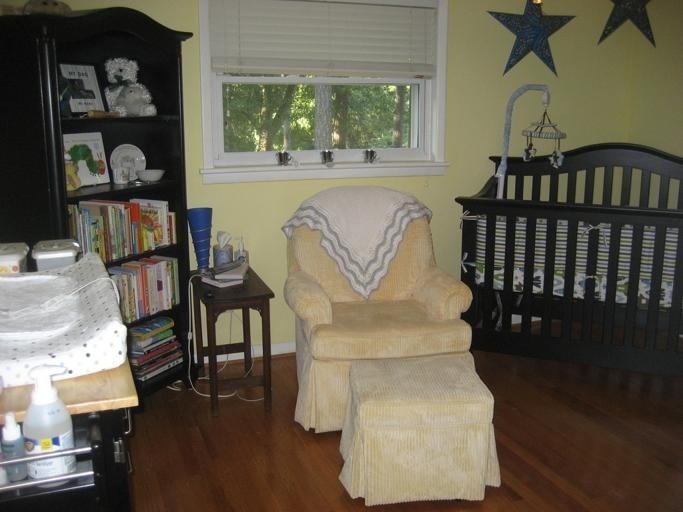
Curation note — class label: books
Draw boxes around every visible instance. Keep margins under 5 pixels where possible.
[128,318,183,381]
[59,61,105,113]
[68,198,177,262]
[106,255,181,324]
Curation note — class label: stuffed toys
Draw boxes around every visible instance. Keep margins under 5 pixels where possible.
[103,57,157,116]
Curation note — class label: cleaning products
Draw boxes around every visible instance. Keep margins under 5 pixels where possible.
[23,365,77,487]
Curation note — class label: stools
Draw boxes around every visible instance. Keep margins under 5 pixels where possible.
[186,264,276,415]
[338,357,494,505]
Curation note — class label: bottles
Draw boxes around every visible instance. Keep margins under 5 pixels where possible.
[2,413,28,483]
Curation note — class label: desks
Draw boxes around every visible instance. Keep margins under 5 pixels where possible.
[1,264,139,512]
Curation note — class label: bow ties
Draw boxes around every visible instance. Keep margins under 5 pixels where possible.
[108,80,129,90]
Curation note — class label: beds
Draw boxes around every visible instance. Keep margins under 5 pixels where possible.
[454,142,683,375]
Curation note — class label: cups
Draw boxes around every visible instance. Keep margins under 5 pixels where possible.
[319,149,334,164]
[275,150,292,166]
[362,148,376,163]
[113,166,130,184]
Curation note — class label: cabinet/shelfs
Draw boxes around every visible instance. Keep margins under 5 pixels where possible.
[0,6,195,401]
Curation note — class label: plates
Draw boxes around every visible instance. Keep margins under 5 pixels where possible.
[109,143,147,180]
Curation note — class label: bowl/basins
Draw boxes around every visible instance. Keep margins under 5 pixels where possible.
[136,168,166,182]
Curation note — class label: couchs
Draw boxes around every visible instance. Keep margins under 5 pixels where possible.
[283,187,473,433]
